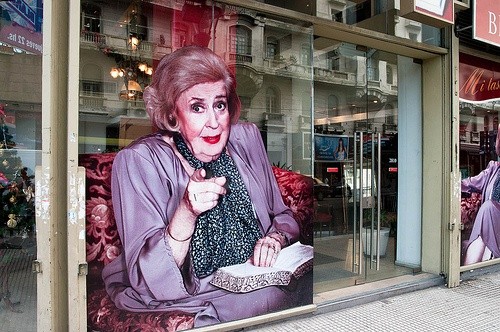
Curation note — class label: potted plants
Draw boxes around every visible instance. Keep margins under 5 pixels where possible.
[360,208,396,260]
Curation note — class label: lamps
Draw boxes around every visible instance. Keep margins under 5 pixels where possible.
[101,7,156,100]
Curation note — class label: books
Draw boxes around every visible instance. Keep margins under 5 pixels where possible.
[208,239,314,294]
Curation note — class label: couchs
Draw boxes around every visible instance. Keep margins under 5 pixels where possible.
[79,149,312,331]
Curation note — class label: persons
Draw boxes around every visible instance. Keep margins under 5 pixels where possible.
[101,45,314,329]
[333,137,347,161]
[460,121,499,265]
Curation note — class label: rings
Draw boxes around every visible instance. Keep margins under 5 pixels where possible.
[193,193,197,202]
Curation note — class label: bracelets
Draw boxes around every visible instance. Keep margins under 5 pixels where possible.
[166,228,193,242]
